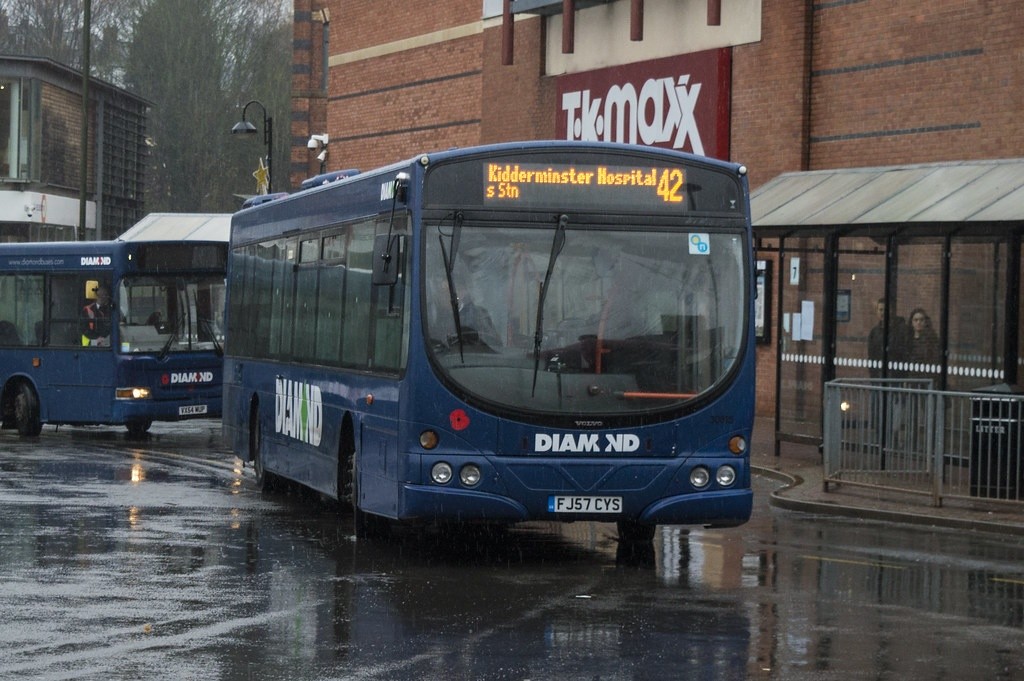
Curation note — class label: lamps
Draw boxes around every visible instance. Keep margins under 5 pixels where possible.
[317,150,327,162]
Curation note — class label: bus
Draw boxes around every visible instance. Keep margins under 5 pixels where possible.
[0,212,238,440]
[220,137,761,549]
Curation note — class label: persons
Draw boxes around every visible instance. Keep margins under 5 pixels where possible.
[79,286,127,347]
[868,298,910,401]
[904,308,945,413]
[431,275,504,348]
[583,281,664,368]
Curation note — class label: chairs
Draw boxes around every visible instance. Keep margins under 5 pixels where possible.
[0,320,22,346]
[34,320,65,345]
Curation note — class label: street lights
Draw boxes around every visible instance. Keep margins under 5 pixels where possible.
[230,100,274,197]
